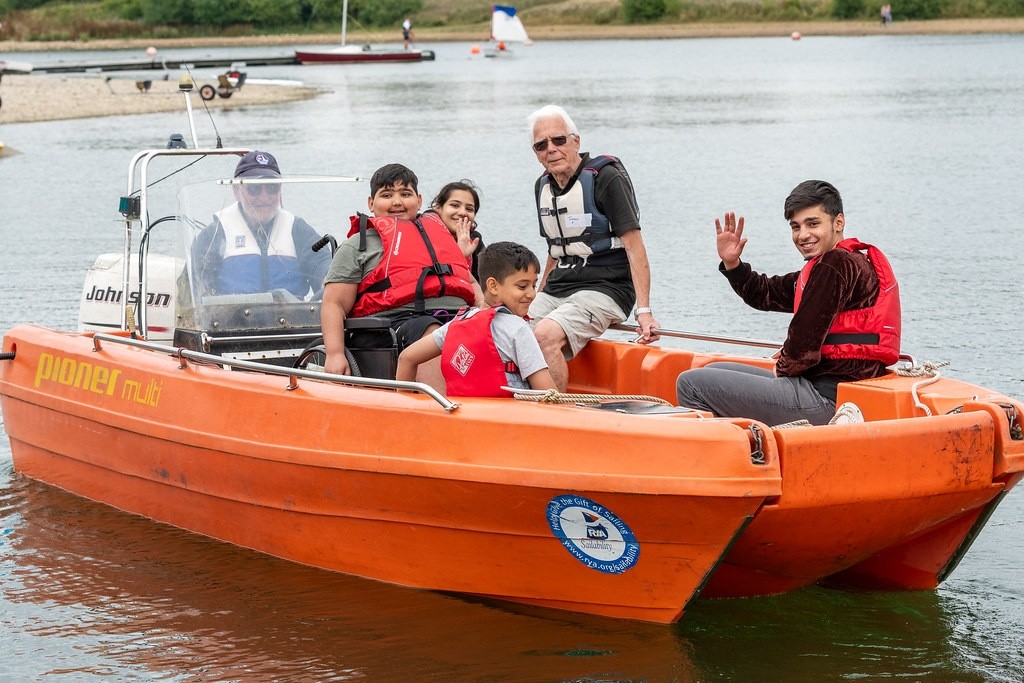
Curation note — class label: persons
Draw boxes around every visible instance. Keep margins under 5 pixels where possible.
[320,164,486,378]
[428,178,486,289]
[880,3,890,28]
[524,105,662,393]
[393,241,561,398]
[175,149,332,330]
[676,180,902,436]
[402,15,415,50]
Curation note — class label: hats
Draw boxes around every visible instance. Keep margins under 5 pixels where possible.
[234,149,281,179]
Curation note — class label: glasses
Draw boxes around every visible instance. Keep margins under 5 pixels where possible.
[532,133,576,151]
[238,183,279,196]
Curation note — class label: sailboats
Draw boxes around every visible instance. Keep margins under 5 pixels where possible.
[481,0,534,58]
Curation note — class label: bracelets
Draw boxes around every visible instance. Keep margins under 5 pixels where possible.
[634,307,653,320]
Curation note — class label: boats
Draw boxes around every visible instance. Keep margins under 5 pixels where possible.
[293,0,436,63]
[0,84,1024,627]
